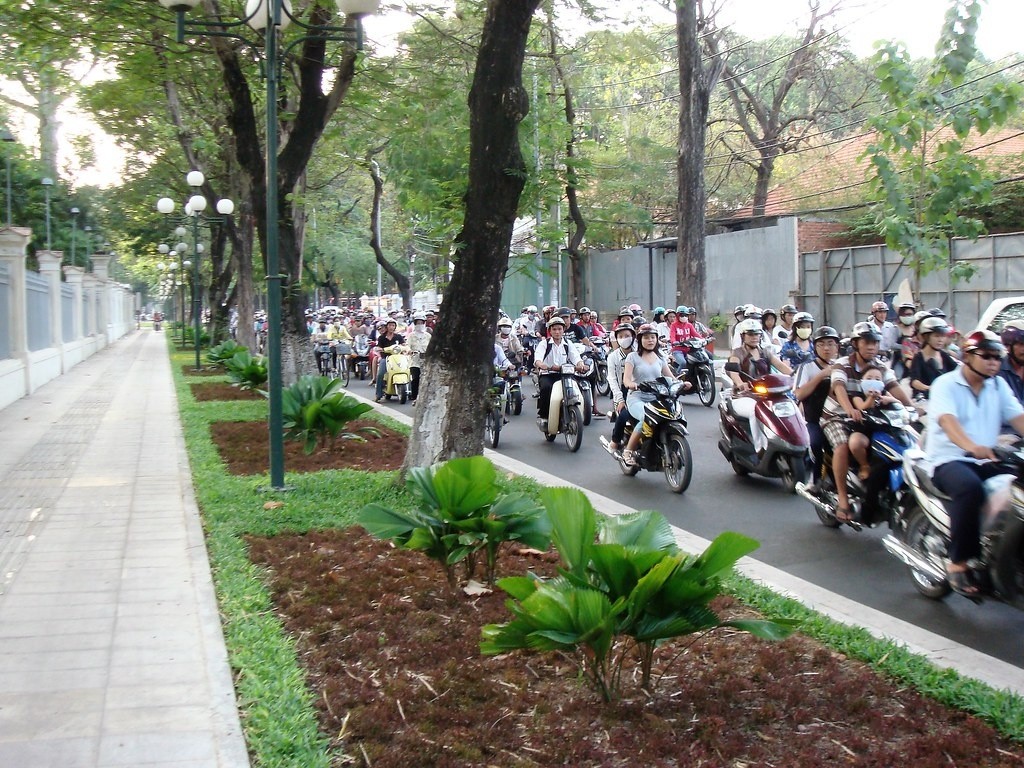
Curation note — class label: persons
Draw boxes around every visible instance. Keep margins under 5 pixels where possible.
[154,301,1022,601]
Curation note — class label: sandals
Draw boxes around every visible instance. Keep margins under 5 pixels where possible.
[621,449,637,465]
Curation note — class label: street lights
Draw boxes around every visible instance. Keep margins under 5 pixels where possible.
[157,171,234,373]
[41,177,54,251]
[160,0,381,492]
[157,227,205,349]
[71,207,80,266]
[84,225,92,274]
[2,132,16,226]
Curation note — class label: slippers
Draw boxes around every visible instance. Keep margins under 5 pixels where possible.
[945,564,980,597]
[835,505,853,524]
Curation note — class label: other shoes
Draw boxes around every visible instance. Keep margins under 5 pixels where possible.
[858,466,871,480]
[609,440,617,453]
[374,392,383,402]
[541,419,548,427]
[369,380,374,385]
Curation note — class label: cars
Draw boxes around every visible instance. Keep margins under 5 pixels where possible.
[976,296,1024,335]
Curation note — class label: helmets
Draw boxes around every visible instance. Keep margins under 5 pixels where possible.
[963,329,1004,352]
[919,317,955,335]
[931,308,947,316]
[1001,326,1024,345]
[913,310,932,323]
[311,302,916,343]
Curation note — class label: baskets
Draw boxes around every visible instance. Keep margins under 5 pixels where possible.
[337,344,352,355]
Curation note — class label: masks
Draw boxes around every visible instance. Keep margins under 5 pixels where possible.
[901,316,913,325]
[618,337,633,349]
[500,328,512,334]
[860,380,885,392]
[795,327,812,339]
[679,317,688,322]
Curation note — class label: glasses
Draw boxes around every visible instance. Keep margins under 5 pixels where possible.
[692,313,695,316]
[680,314,688,317]
[972,351,1001,361]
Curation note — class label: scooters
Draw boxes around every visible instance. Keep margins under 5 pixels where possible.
[599,369,693,493]
[718,331,1024,611]
[313,314,716,452]
